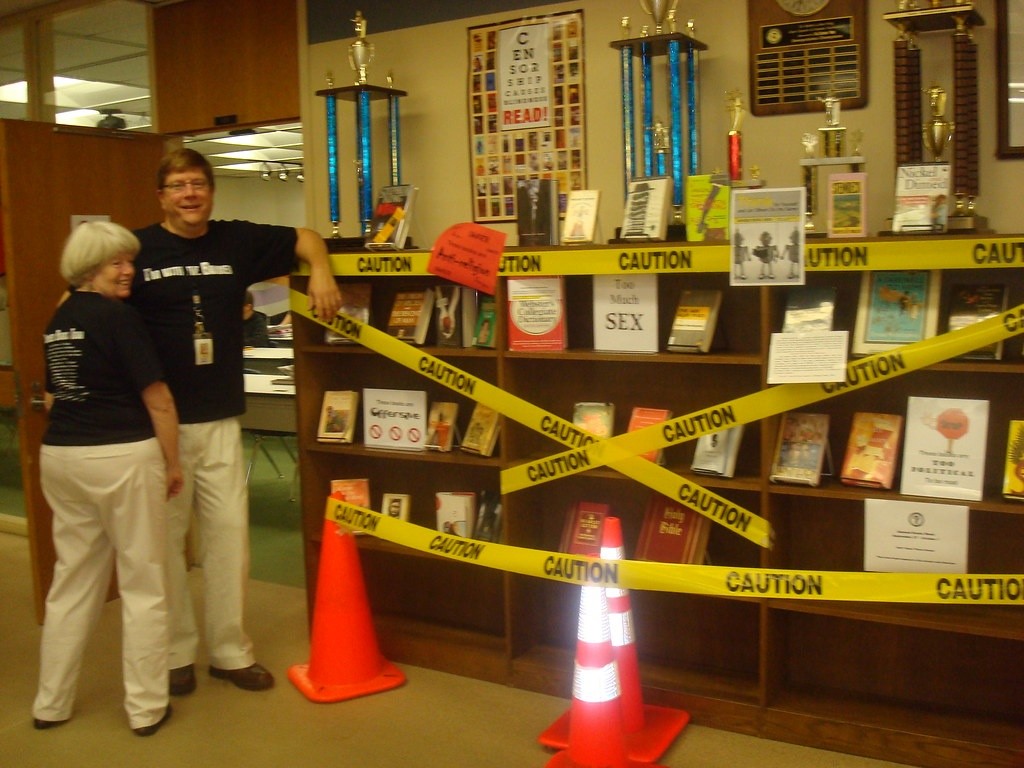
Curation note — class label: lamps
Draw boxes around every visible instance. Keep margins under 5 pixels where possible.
[260,162,305,183]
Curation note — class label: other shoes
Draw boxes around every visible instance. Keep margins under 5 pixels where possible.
[137,717,164,737]
[34,718,50,728]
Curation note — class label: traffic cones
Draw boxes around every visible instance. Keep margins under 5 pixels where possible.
[545,553,667,768]
[538,515,691,765]
[289,491,408,704]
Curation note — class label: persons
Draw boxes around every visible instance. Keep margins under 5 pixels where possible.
[618,178,675,241]
[30,219,181,736]
[49,146,343,696]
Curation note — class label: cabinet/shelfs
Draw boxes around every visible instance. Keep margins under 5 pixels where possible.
[289,233,1024,767]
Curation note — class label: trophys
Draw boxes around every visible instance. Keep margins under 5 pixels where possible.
[724,88,744,134]
[649,121,673,154]
[346,8,375,85]
[921,86,956,164]
[638,0,679,36]
[816,94,843,128]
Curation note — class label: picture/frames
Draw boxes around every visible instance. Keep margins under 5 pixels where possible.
[996,0,1024,161]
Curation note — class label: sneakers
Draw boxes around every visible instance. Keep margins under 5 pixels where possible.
[166,663,195,695]
[210,659,273,691]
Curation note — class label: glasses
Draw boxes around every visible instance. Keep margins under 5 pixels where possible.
[160,178,212,193]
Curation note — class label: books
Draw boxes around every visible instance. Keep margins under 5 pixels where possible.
[470,299,498,349]
[516,179,559,245]
[506,274,568,352]
[384,289,435,345]
[366,185,420,250]
[593,273,660,353]
[381,494,411,523]
[460,398,501,456]
[426,403,458,452]
[322,282,371,344]
[620,407,673,466]
[572,403,613,441]
[890,164,952,235]
[435,491,475,538]
[666,288,724,352]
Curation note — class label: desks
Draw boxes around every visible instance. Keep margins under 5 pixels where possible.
[242,345,297,435]
